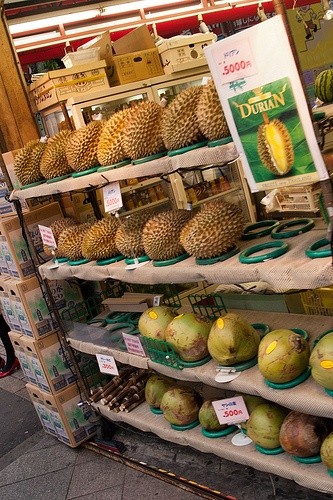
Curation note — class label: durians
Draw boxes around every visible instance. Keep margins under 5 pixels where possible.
[13,81,231,186]
[257,113,294,176]
[43,200,248,260]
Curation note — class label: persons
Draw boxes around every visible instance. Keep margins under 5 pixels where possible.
[0,308,21,378]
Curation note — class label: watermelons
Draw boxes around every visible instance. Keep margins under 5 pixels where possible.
[314,68,333,103]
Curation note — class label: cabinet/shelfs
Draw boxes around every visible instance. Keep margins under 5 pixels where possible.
[0,103,333,496]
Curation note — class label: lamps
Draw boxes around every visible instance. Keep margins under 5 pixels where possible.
[257,3,267,21]
[320,0,333,20]
[151,23,164,43]
[197,14,209,33]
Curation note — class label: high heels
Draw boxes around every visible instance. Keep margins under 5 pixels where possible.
[0,357,5,370]
[0,357,21,378]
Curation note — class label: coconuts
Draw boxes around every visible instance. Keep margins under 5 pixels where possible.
[139,305,333,389]
[146,372,333,472]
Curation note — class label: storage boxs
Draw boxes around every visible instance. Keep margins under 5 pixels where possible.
[157,34,214,75]
[75,24,164,87]
[0,149,125,448]
[211,284,333,317]
[29,59,110,112]
[273,187,322,213]
